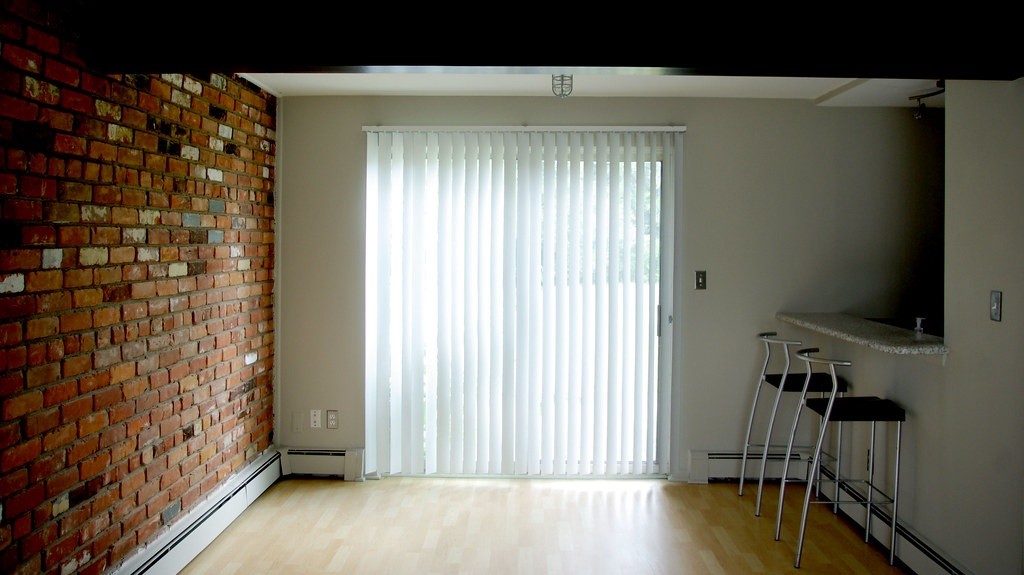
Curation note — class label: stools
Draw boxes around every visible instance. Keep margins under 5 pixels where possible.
[774,345,907,564]
[737,331,849,518]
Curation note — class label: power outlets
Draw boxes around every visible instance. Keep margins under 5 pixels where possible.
[292,410,303,432]
[327,410,338,429]
[309,409,321,428]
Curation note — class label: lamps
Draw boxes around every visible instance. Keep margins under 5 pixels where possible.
[551,74,575,99]
[907,88,945,119]
